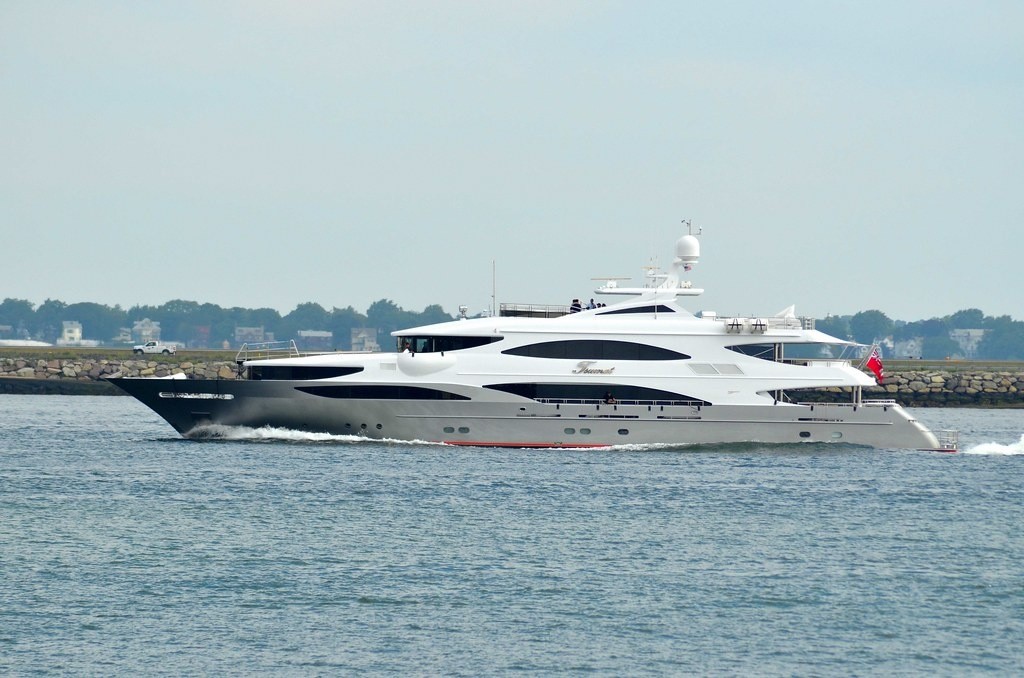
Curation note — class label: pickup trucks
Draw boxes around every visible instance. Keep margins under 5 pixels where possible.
[132,340,176,356]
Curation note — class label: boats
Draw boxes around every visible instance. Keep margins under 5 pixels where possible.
[90,220,965,454]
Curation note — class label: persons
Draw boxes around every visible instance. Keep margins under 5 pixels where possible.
[569,298,606,314]
[403,343,414,353]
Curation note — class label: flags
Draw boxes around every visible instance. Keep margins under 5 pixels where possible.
[866,348,885,384]
